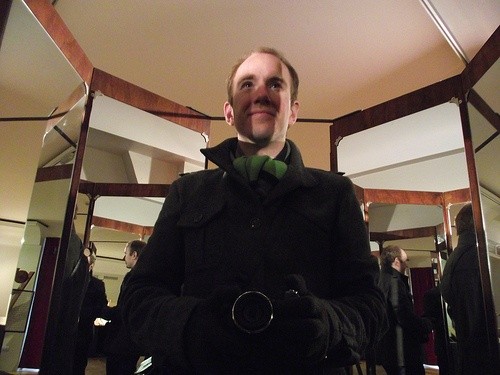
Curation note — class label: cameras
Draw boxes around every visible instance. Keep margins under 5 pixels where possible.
[220,290,300,346]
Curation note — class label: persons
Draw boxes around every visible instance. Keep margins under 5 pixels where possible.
[39,204,500,375]
[115,47,390,375]
